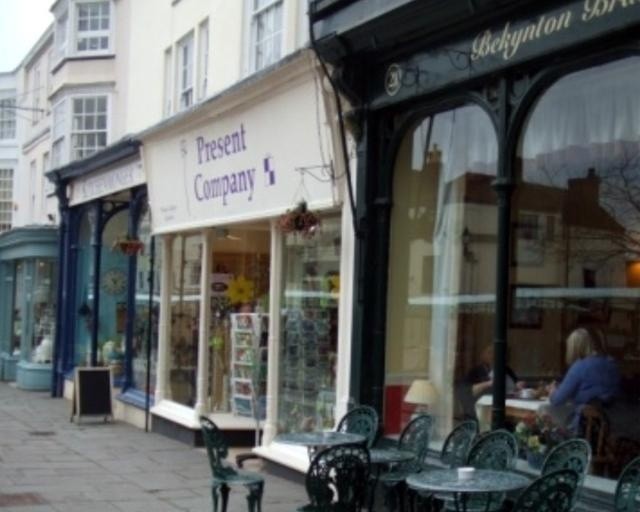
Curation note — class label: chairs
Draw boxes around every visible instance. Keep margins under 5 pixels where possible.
[615,458,640,512]
[454,429,520,512]
[299,442,371,512]
[199,415,264,512]
[334,406,379,450]
[539,439,592,499]
[514,470,580,512]
[416,421,479,512]
[581,408,618,477]
[378,414,431,512]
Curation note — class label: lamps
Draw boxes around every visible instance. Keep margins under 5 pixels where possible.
[402,380,438,421]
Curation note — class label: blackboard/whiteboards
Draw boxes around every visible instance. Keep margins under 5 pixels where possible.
[73,367,115,417]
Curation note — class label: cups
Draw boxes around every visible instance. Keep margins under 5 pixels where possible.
[456,467,475,479]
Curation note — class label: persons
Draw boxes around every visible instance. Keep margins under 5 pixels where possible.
[546,328,619,455]
[454,339,528,421]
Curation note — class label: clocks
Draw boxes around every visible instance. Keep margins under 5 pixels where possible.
[99,268,129,294]
